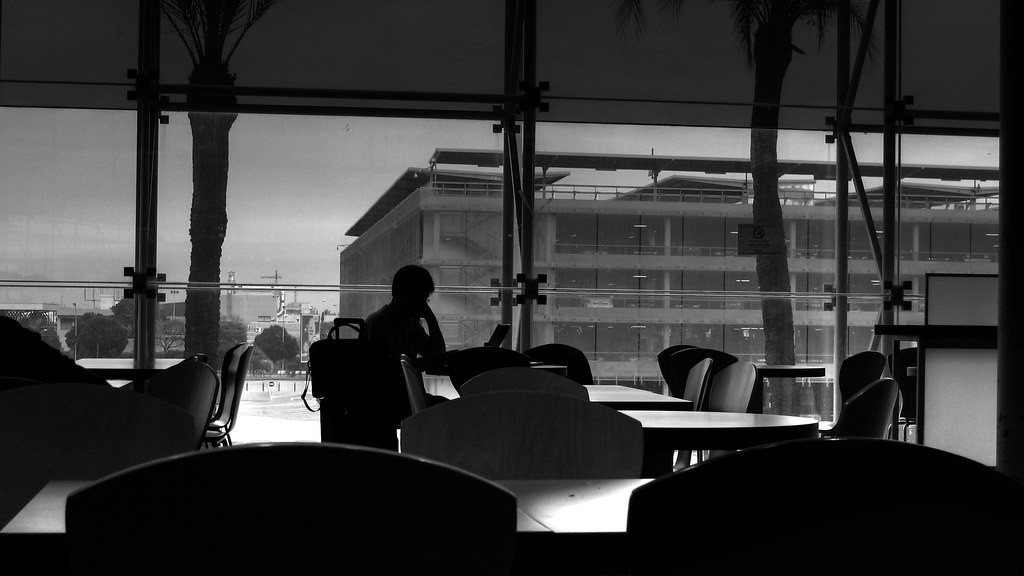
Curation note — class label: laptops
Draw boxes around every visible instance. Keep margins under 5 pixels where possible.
[485,324,511,348]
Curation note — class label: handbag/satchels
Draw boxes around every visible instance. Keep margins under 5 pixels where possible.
[302,324,370,412]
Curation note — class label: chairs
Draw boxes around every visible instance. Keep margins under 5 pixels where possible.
[0,339,1022,573]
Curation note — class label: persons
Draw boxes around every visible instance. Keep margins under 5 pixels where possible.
[357,265,451,422]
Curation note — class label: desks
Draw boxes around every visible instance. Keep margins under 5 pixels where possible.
[0,479,553,543]
[581,383,695,411]
[614,409,821,451]
[493,476,661,539]
[70,357,186,381]
[423,366,568,379]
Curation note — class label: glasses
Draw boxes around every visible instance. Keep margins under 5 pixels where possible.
[425,297,430,303]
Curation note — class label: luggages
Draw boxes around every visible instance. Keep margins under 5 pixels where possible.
[320,318,366,443]
